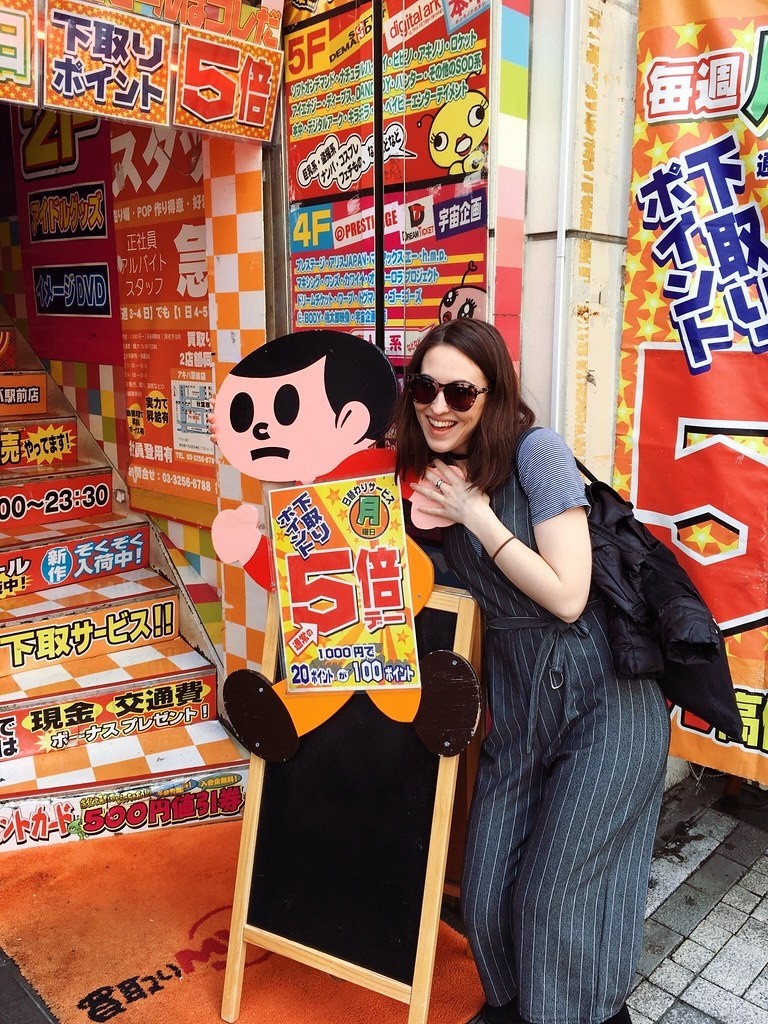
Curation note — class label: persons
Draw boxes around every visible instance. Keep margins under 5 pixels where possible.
[395,318,675,1024]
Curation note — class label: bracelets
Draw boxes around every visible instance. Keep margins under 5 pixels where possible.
[491,535,517,562]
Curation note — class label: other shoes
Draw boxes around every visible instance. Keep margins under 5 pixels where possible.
[599,1002,633,1024]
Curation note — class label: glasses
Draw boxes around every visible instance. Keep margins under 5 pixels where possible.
[406,374,491,413]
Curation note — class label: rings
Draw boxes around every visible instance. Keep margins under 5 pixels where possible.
[436,478,445,488]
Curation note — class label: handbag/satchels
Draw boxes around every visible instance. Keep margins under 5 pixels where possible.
[514,427,744,738]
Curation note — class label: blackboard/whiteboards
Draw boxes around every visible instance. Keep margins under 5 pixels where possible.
[231,567,480,1004]
